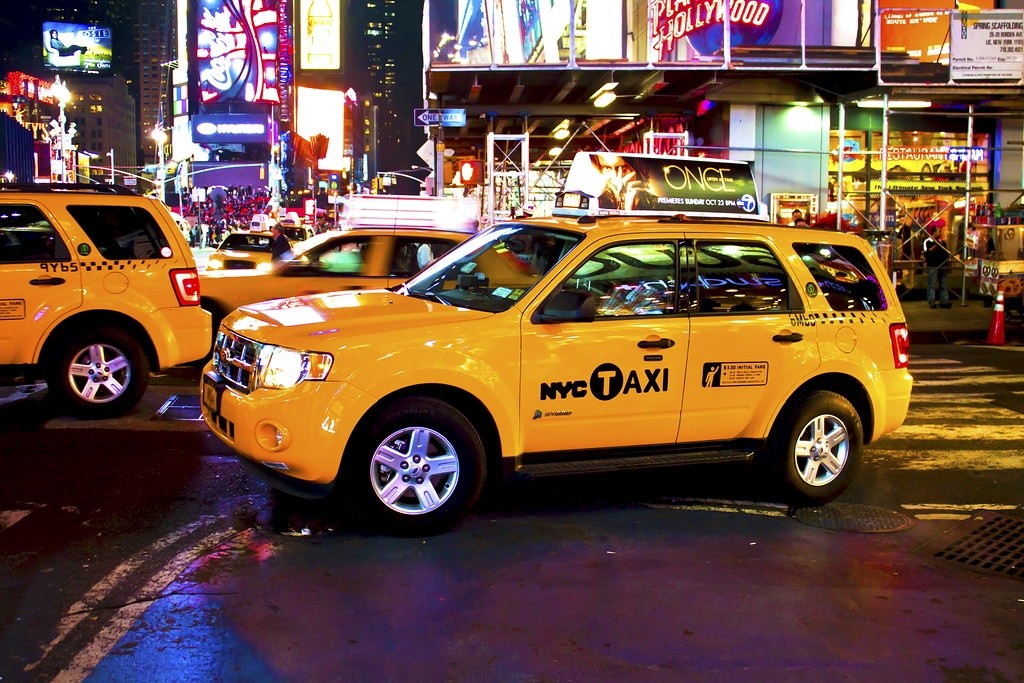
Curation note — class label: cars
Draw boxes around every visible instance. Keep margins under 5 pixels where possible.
[275,212,318,269]
[188,193,545,371]
[375,172,429,197]
[204,214,312,272]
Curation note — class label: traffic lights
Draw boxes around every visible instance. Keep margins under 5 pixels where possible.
[460,160,486,185]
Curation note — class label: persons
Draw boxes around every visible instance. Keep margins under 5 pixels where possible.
[923,226,950,308]
[270,223,291,261]
[788,210,809,227]
[589,155,673,211]
[189,184,332,248]
[50,29,87,54]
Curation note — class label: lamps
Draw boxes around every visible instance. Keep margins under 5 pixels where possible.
[590,81,619,107]
[551,118,571,139]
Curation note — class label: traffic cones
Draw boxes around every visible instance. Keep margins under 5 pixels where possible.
[981,284,1011,347]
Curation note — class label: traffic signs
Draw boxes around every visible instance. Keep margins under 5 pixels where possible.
[414,108,467,128]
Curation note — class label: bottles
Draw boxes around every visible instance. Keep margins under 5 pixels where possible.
[976,202,1001,218]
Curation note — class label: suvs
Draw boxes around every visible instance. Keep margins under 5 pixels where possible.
[0,169,213,420]
[199,150,914,540]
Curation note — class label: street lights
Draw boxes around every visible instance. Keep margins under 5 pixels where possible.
[151,129,167,204]
[106,148,114,185]
[50,82,75,182]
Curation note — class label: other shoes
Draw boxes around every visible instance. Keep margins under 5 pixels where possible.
[930,304,937,309]
[939,303,952,309]
